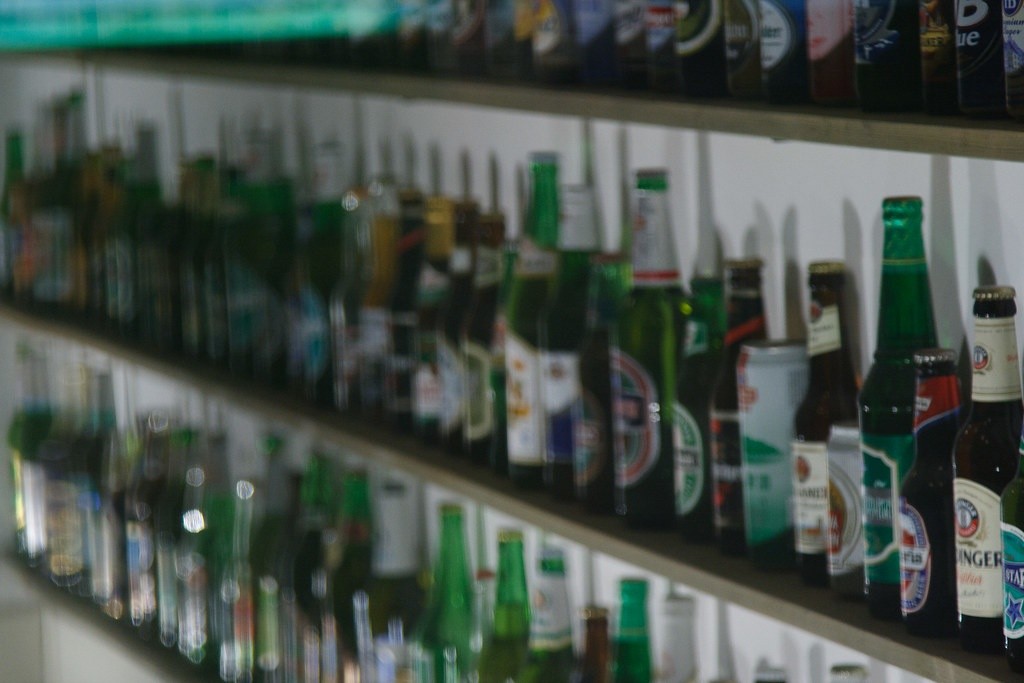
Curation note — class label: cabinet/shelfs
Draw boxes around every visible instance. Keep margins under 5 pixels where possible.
[0,42,1024,683]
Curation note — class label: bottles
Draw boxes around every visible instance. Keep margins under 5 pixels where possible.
[0,0,1024,683]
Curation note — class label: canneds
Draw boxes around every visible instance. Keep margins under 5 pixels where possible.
[735,340,812,569]
